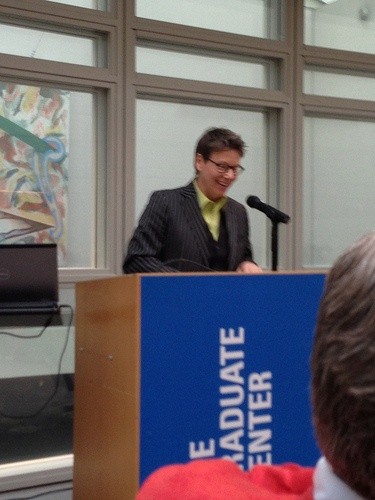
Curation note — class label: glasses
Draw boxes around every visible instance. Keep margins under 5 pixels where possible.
[202,154,245,176]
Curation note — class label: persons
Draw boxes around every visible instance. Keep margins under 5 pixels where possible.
[135,229,374,499]
[121,128,267,273]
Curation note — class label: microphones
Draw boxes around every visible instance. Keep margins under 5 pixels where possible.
[246,196,290,225]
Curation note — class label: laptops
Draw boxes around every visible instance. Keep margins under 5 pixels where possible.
[0,243,62,325]
